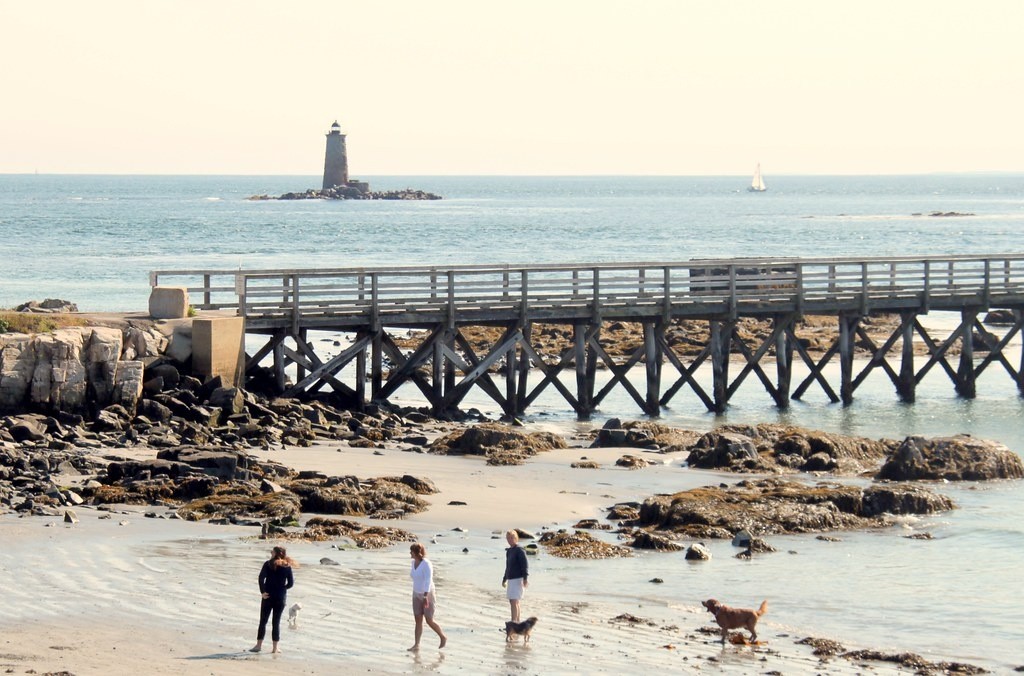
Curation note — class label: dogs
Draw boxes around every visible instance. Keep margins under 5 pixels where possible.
[286,602,303,626]
[701,597,769,645]
[504,616,539,644]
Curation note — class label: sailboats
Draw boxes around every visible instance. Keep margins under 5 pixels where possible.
[748,163,767,192]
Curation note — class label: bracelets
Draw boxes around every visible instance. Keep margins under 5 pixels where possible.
[424,596,427,598]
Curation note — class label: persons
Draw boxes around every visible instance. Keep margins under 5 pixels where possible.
[407,543,447,650]
[502,529,528,636]
[249,546,294,653]
